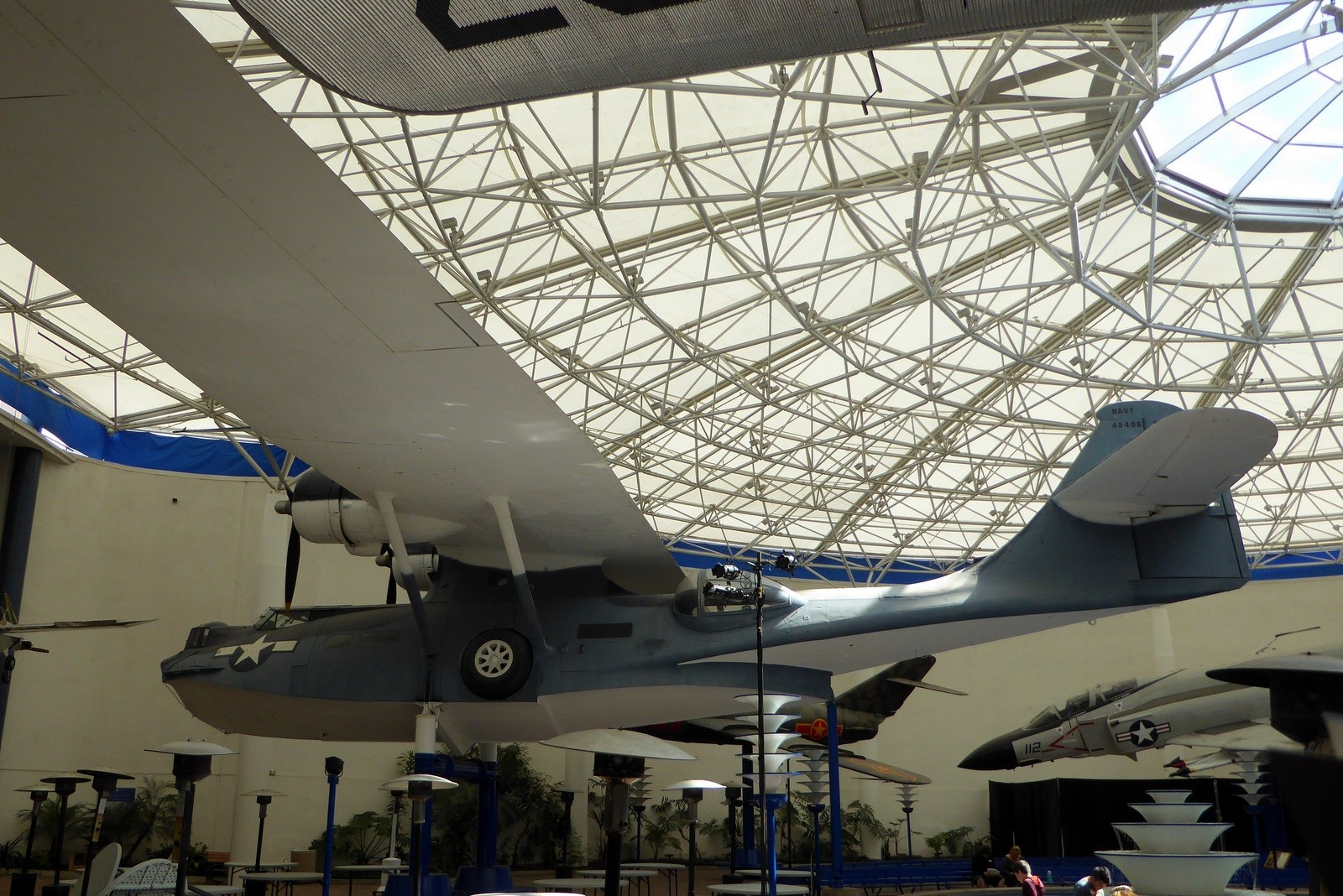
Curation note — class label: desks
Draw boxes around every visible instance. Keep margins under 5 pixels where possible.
[239,872,324,896]
[620,863,685,896]
[60,879,79,892]
[532,879,629,896]
[334,865,408,896]
[224,862,297,896]
[706,882,810,896]
[576,870,658,896]
[734,869,816,896]
[188,886,245,896]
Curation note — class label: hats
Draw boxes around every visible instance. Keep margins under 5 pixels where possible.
[1110,885,1132,894]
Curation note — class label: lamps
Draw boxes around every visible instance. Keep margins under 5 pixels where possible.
[8,693,829,896]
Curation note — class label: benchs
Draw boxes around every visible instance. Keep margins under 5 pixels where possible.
[796,858,1131,896]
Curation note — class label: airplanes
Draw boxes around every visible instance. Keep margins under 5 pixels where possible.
[1,1,1343,787]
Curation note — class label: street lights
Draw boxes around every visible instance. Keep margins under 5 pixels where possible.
[703,548,802,896]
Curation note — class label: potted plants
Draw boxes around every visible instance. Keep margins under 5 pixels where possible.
[926,826,990,858]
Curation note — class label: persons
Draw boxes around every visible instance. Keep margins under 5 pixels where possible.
[1012,860,1046,896]
[971,846,1005,888]
[1072,866,1111,896]
[1000,846,1023,887]
[1111,885,1137,896]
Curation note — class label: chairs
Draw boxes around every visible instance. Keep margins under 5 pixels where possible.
[97,858,188,896]
[70,842,121,896]
